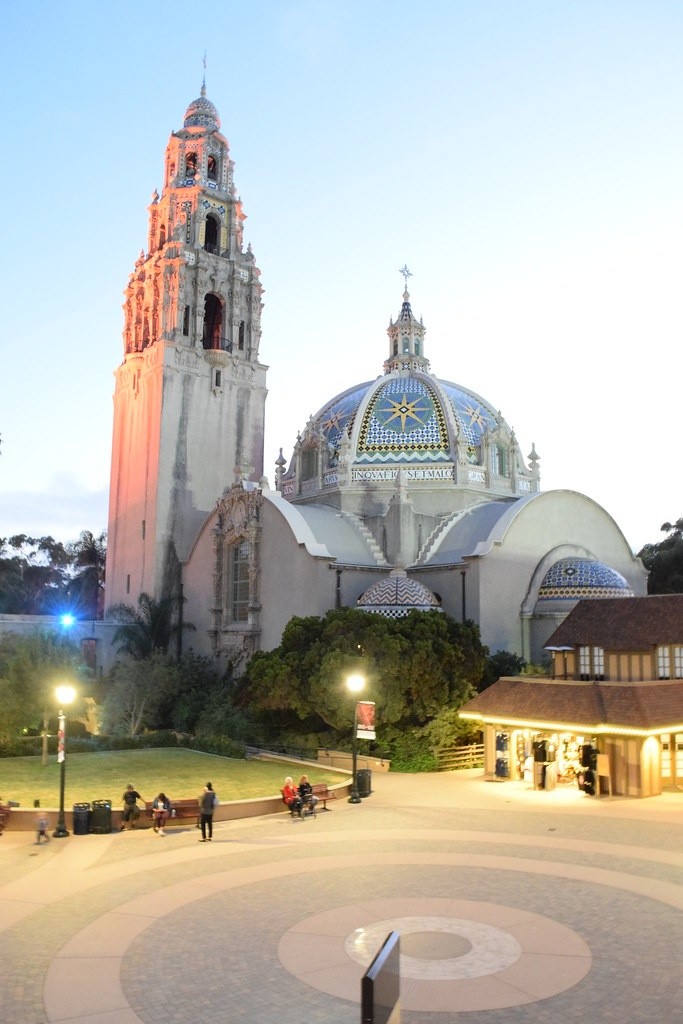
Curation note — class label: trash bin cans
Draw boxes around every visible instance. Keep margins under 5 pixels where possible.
[355,768,372,797]
[92,799,113,834]
[71,802,91,835]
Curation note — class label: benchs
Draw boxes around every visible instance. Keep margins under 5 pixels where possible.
[145,799,202,832]
[280,784,337,817]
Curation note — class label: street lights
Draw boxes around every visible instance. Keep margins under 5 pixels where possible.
[53,683,76,839]
[346,672,367,805]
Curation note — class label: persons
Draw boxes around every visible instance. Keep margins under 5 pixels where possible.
[198,782,217,843]
[34,812,50,844]
[119,784,148,830]
[300,776,319,814]
[283,777,302,818]
[151,792,172,836]
